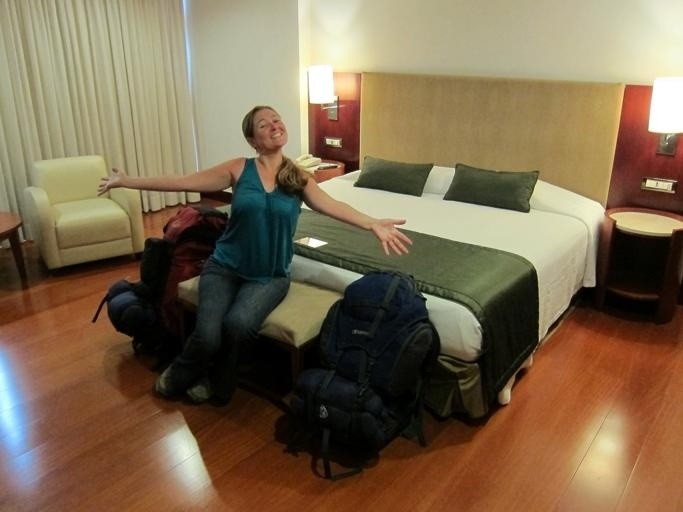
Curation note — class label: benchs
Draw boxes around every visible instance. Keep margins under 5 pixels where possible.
[176,269,344,409]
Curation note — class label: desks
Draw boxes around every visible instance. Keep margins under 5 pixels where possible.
[0,212,29,281]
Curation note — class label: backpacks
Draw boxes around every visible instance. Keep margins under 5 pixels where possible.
[283,269,441,470]
[103,201,232,375]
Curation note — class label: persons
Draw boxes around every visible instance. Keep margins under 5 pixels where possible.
[97,105,413,408]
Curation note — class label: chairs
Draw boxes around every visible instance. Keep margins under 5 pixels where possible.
[25,152,145,275]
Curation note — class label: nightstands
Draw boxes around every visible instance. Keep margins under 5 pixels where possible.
[591,207,682,326]
[298,156,345,182]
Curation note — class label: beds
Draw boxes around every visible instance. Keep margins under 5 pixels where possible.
[217,69,626,421]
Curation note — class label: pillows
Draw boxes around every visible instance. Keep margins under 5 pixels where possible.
[442,162,539,211]
[353,153,433,198]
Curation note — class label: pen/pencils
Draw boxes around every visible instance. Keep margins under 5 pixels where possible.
[317,165,339,170]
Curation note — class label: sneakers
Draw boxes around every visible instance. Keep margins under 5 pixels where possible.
[187,373,210,398]
[155,358,184,399]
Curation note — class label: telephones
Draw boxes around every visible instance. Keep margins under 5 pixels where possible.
[295,153,322,167]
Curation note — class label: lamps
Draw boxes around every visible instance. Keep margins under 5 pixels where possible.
[306,63,338,122]
[646,74,683,157]
